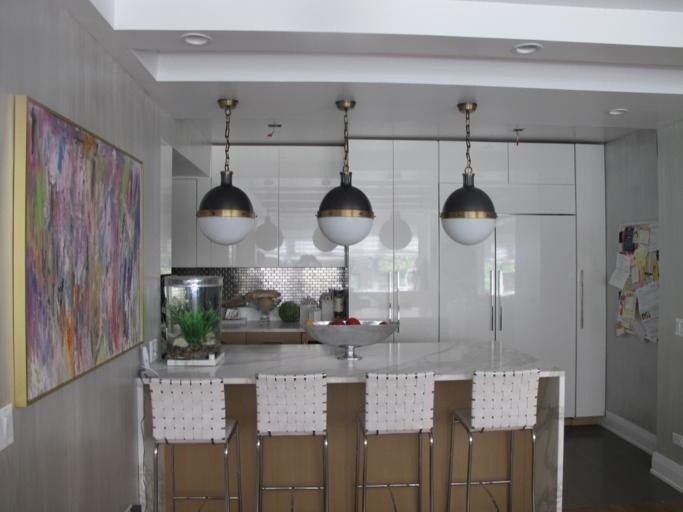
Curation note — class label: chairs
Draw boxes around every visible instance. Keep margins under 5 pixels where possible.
[148,376,243,511]
[254,373,329,512]
[447,369,540,512]
[353,371,435,512]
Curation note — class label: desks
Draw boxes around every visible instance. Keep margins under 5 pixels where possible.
[146,342,565,512]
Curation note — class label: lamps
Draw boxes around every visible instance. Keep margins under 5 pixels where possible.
[315,99,375,246]
[196,98,258,247]
[441,103,498,246]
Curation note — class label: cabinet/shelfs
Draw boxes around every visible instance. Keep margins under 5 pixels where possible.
[173,139,608,422]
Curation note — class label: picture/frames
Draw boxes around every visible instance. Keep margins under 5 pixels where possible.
[14,94,144,409]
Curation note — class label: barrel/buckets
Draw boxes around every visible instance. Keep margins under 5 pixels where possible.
[164,276,222,360]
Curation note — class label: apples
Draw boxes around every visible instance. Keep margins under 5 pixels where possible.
[346,318,360,325]
[329,320,344,325]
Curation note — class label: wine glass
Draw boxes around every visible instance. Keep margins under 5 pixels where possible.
[248,298,282,324]
